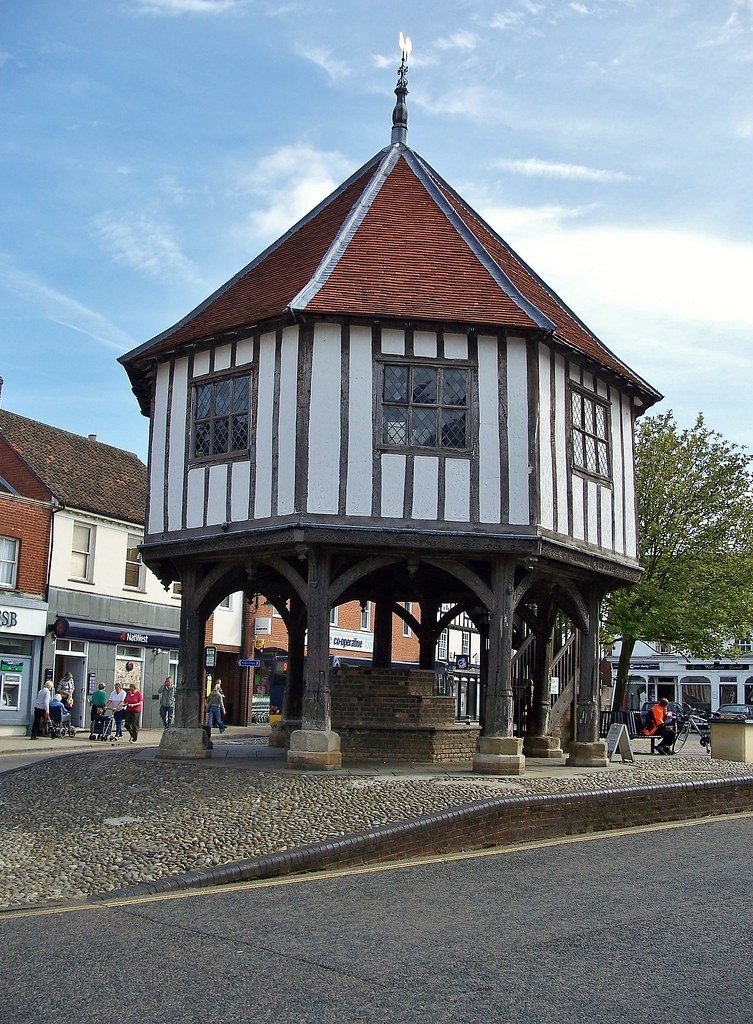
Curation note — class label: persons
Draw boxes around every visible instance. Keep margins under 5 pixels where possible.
[56,672,75,711]
[205,684,226,734]
[158,677,176,729]
[30,681,58,740]
[104,682,127,740]
[642,698,675,755]
[122,683,143,743]
[213,679,228,729]
[49,694,75,733]
[89,682,106,740]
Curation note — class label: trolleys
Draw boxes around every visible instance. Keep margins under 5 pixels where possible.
[88,707,116,742]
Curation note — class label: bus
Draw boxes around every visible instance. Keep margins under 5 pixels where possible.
[270,655,456,726]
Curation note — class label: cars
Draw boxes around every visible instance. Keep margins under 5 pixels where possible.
[713,704,753,720]
[639,702,711,731]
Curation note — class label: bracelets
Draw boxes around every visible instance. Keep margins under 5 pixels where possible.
[64,691,66,694]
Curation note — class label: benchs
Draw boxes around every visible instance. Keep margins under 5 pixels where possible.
[600,710,663,755]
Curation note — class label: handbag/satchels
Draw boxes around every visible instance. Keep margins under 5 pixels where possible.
[205,705,211,713]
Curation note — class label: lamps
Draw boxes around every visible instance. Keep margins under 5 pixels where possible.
[714,661,720,666]
[153,647,162,655]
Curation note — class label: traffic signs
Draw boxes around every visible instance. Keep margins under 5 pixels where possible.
[238,660,260,667]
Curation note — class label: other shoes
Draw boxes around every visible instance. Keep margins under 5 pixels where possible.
[118,736,122,739]
[220,728,225,733]
[128,736,133,742]
[30,736,38,740]
[654,745,667,755]
[131,738,137,744]
[663,746,674,755]
[114,736,118,740]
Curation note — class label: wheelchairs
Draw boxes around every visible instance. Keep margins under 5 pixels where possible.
[44,705,76,739]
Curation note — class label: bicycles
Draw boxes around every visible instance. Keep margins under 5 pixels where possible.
[672,701,711,754]
